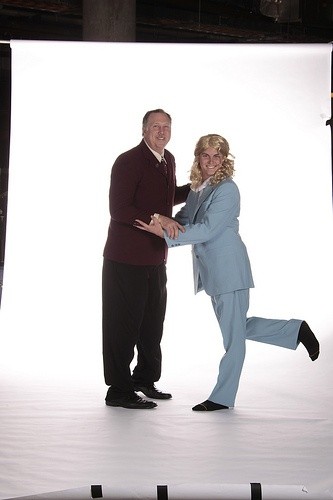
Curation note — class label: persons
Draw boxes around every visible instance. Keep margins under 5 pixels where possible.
[101,109,191,409]
[133,134,320,411]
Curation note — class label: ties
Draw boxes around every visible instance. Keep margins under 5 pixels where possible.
[157,156,168,169]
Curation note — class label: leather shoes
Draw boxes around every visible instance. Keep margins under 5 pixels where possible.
[128,374,173,399]
[104,387,156,410]
